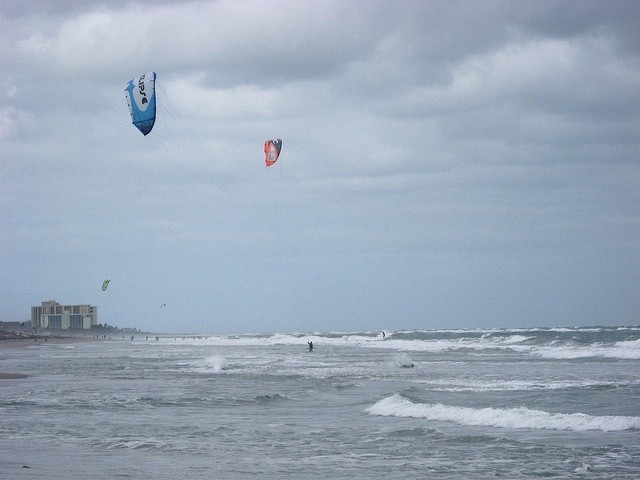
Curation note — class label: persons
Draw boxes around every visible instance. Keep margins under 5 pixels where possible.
[308,342,313,352]
